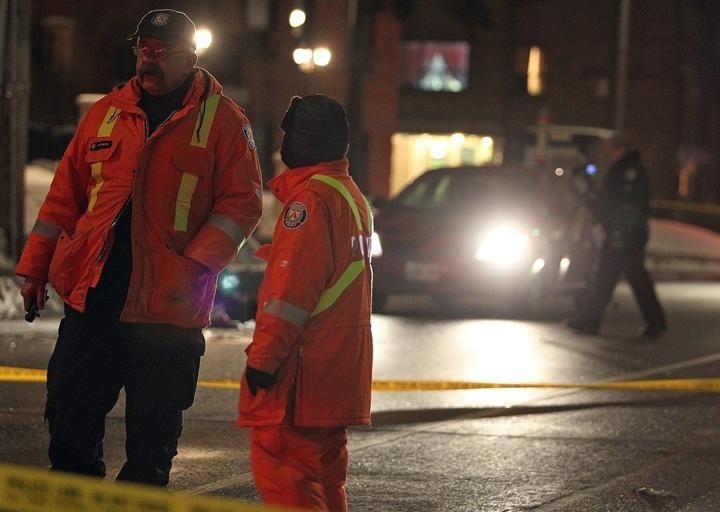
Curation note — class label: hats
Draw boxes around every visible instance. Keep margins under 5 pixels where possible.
[127,8,197,48]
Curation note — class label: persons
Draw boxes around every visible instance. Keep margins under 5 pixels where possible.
[9,8,264,489]
[563,128,673,342]
[235,94,378,511]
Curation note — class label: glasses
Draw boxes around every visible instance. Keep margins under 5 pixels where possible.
[131,44,190,57]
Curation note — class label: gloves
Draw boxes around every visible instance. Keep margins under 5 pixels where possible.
[245,363,278,396]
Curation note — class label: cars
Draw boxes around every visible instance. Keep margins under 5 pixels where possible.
[365,162,612,323]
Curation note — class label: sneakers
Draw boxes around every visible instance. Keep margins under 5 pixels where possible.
[645,316,667,334]
[563,315,600,334]
